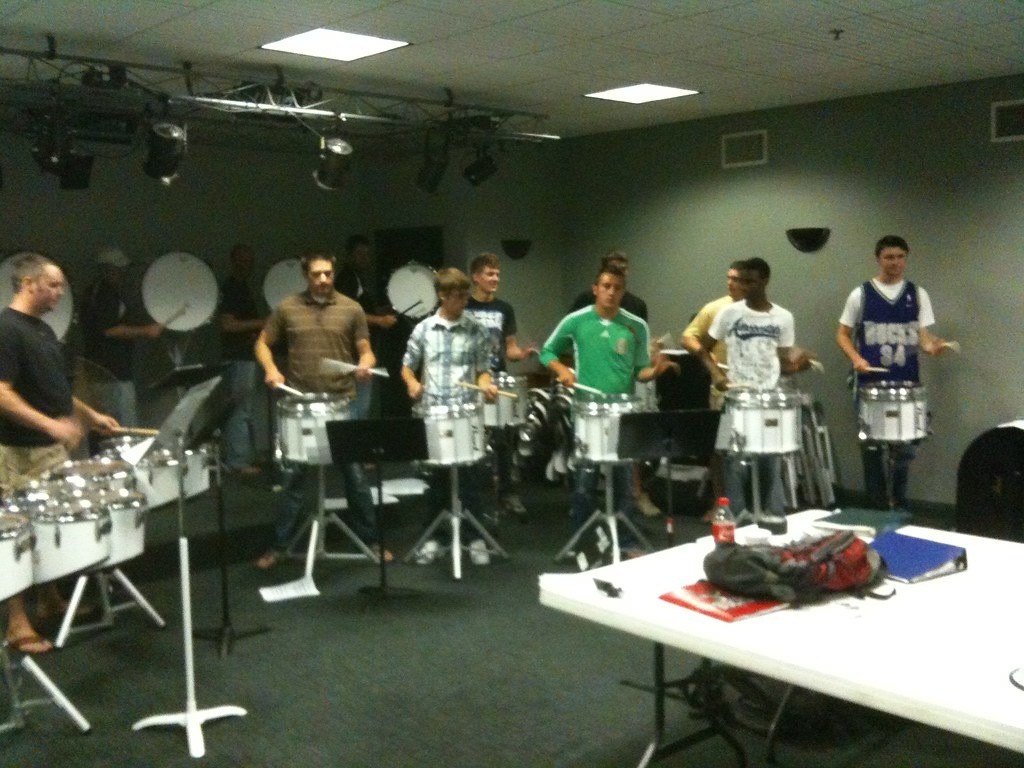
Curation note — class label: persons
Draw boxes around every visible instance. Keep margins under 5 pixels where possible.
[252,247,395,571]
[82,235,397,479]
[0,252,121,656]
[400,249,819,567]
[836,236,947,522]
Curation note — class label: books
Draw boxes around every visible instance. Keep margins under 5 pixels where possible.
[812,506,914,536]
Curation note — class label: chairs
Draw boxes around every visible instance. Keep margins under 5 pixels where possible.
[956,427,1024,540]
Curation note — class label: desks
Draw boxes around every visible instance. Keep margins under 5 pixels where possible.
[539,540,1024,768]
[694,507,1024,623]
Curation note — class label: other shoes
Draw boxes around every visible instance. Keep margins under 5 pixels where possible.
[256,549,281,569]
[369,544,393,563]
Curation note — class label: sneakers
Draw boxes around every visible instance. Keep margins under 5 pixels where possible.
[503,493,526,518]
[416,540,439,565]
[467,539,491,566]
[635,492,662,518]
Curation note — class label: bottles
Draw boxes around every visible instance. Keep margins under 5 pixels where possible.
[710,497,735,549]
[757,515,789,547]
[742,527,772,548]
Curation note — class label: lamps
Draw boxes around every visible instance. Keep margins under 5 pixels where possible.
[57,149,95,191]
[312,138,354,192]
[145,122,187,180]
[462,154,499,187]
[415,154,451,195]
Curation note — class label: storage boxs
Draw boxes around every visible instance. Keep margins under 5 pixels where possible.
[651,462,716,517]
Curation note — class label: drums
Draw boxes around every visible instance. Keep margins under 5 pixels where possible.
[262,256,310,313]
[0,434,213,603]
[858,380,929,442]
[574,393,645,464]
[723,388,803,455]
[385,260,441,319]
[412,400,488,469]
[483,374,530,429]
[0,250,82,345]
[274,391,352,467]
[140,249,220,333]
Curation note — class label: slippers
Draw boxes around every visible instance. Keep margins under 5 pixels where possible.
[5,634,55,658]
[35,599,91,621]
